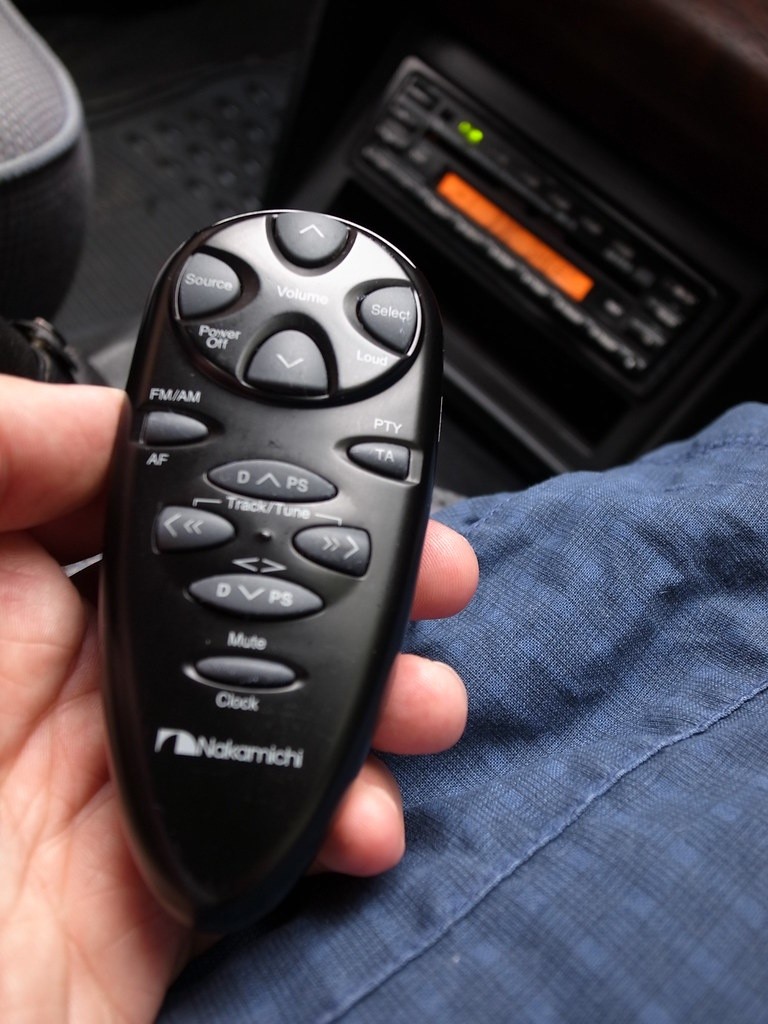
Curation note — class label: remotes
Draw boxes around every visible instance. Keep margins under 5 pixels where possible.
[96,206,447,944]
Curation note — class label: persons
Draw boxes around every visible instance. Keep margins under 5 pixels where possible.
[4,370,767,1022]
